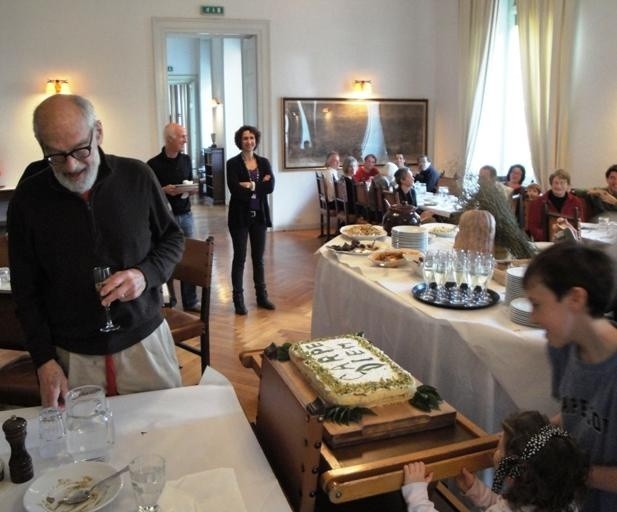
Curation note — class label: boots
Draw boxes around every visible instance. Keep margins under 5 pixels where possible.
[233,289,248,314]
[254,284,275,309]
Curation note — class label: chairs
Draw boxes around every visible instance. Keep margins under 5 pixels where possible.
[159,234,217,383]
[313,166,585,246]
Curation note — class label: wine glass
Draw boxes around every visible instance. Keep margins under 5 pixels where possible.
[92,265,121,333]
[420,250,495,307]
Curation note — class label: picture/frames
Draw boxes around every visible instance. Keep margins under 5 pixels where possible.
[282,97,429,170]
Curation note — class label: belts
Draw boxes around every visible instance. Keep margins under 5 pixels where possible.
[243,209,263,217]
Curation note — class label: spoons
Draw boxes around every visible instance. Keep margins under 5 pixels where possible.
[66,465,130,505]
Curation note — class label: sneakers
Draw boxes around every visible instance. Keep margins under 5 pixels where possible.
[185,302,202,312]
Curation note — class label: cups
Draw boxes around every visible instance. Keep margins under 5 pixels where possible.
[438,186,449,200]
[128,452,166,512]
[63,384,116,463]
[598,217,609,233]
[37,408,64,460]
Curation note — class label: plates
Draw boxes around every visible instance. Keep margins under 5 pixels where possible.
[424,201,438,206]
[391,225,429,250]
[339,224,387,240]
[22,463,123,512]
[504,267,539,327]
[177,183,199,192]
[368,253,407,268]
[421,223,457,236]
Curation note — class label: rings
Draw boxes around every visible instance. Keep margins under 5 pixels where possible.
[120,292,127,300]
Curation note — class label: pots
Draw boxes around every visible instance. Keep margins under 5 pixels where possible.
[382,198,422,236]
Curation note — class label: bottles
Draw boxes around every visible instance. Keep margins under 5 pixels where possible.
[1,414,34,484]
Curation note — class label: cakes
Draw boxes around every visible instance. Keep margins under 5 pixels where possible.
[288,335,418,409]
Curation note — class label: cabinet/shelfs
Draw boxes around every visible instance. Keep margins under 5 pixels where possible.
[203,147,226,205]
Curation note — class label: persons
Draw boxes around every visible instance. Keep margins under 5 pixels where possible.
[400,410,589,512]
[144,122,202,314]
[519,243,616,511]
[6,94,187,410]
[318,147,617,262]
[226,126,275,315]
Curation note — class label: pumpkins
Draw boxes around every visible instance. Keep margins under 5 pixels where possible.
[454,208,496,258]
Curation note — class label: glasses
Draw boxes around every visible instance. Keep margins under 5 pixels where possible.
[44,129,92,164]
